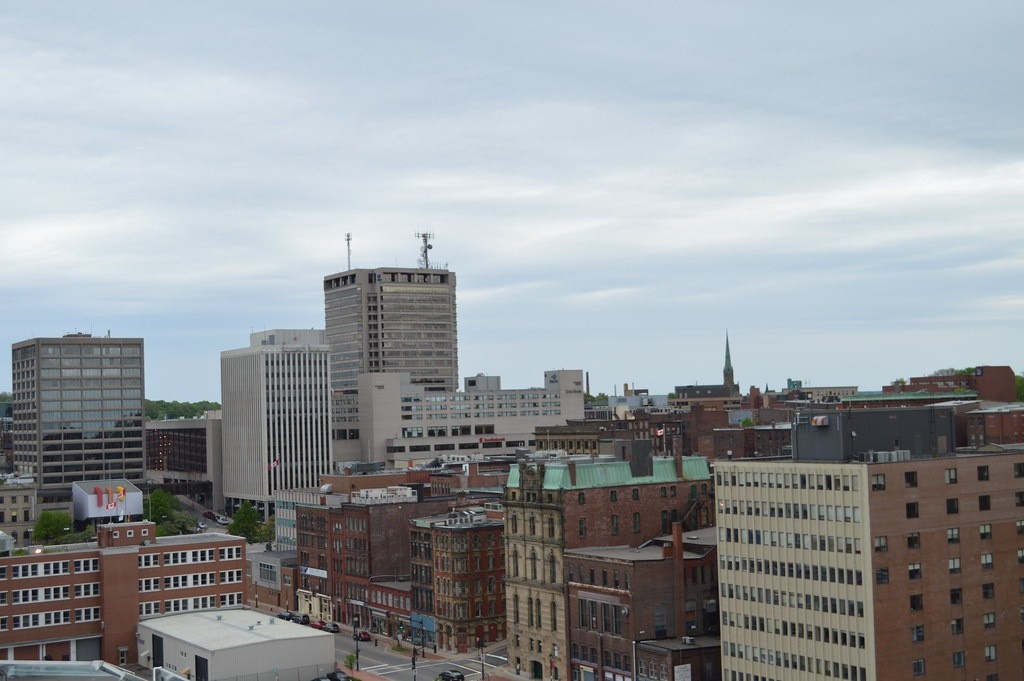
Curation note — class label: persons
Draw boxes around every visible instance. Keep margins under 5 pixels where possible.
[726,448,732,460]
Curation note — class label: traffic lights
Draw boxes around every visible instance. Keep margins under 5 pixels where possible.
[400,621,404,629]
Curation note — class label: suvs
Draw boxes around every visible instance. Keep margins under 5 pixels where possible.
[294,614,309,624]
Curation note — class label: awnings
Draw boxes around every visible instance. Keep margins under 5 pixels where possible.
[139,650,151,656]
[811,416,829,426]
[179,668,191,674]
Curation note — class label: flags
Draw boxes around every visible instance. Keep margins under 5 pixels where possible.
[117,488,126,499]
[106,502,116,510]
[656,429,663,437]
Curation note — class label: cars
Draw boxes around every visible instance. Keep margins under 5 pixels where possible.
[311,621,327,629]
[323,623,340,633]
[197,522,208,532]
[277,611,294,620]
[202,511,230,525]
[353,630,371,641]
[439,670,464,681]
